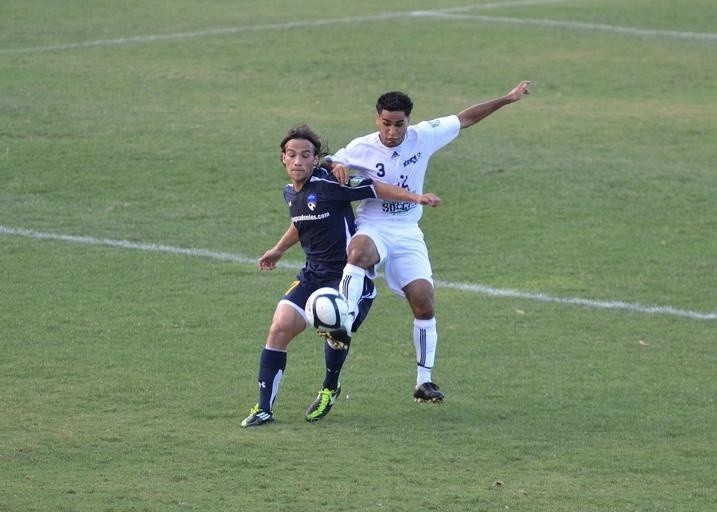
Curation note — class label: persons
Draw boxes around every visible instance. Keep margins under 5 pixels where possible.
[298,79,534,425]
[235,126,440,428]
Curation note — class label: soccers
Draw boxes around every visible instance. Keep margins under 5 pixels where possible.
[305,287,351,334]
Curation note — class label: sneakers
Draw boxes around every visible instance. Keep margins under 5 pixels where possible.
[304,381,341,423]
[414,382,444,403]
[317,326,351,351]
[240,409,274,427]
